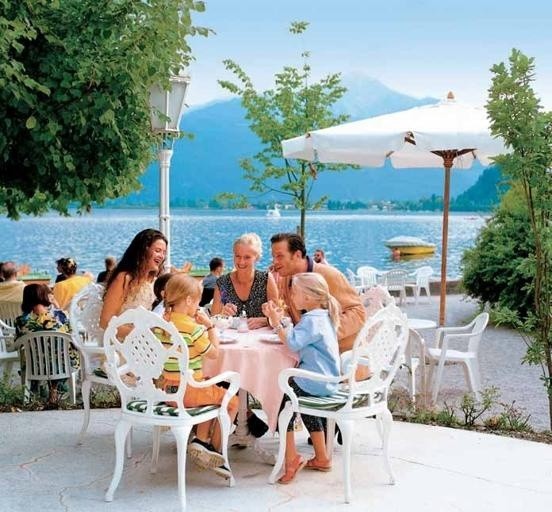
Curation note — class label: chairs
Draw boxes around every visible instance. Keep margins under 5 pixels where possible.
[0,319,22,389]
[13,331,97,409]
[70,283,136,458]
[269,308,407,504]
[347,266,490,419]
[102,309,239,508]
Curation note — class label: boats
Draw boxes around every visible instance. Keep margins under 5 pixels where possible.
[463,215,478,220]
[382,234,438,259]
[268,203,281,216]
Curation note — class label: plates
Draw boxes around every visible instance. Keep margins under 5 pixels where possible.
[264,335,281,343]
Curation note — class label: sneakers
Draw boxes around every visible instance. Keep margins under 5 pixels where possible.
[308,424,339,444]
[189,438,232,479]
[248,414,269,437]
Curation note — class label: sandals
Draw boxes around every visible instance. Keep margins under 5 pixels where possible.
[305,457,331,472]
[278,455,305,483]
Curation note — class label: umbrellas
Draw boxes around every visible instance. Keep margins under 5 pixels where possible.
[280,89,512,329]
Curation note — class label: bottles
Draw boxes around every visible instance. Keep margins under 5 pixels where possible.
[237,316,247,332]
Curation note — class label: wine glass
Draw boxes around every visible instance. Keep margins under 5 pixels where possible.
[212,315,233,343]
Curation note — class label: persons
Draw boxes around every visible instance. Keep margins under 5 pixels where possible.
[2,254,98,410]
[100,230,193,343]
[202,258,223,287]
[211,233,280,438]
[389,243,407,264]
[159,272,240,480]
[151,274,169,313]
[271,233,371,444]
[97,258,115,282]
[261,272,341,482]
[314,249,328,264]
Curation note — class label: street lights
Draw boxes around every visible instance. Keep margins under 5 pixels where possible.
[147,70,190,271]
